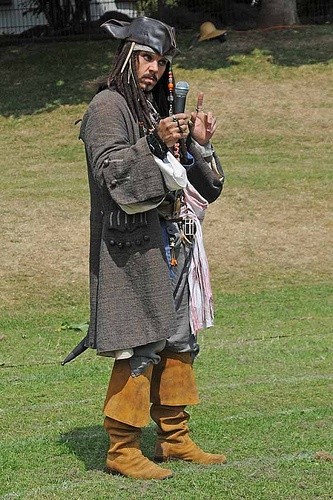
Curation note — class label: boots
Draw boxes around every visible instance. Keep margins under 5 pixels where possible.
[101,357,173,480]
[148,352,226,465]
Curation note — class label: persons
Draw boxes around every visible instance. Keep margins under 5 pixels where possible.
[62,16,227,478]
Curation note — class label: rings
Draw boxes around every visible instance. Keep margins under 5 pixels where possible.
[179,126,182,133]
[195,107,204,111]
[172,115,177,122]
[176,120,180,126]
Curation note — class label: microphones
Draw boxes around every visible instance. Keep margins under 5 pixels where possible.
[173,81,190,114]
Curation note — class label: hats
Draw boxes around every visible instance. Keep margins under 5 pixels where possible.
[99,16,180,59]
[198,22,226,42]
